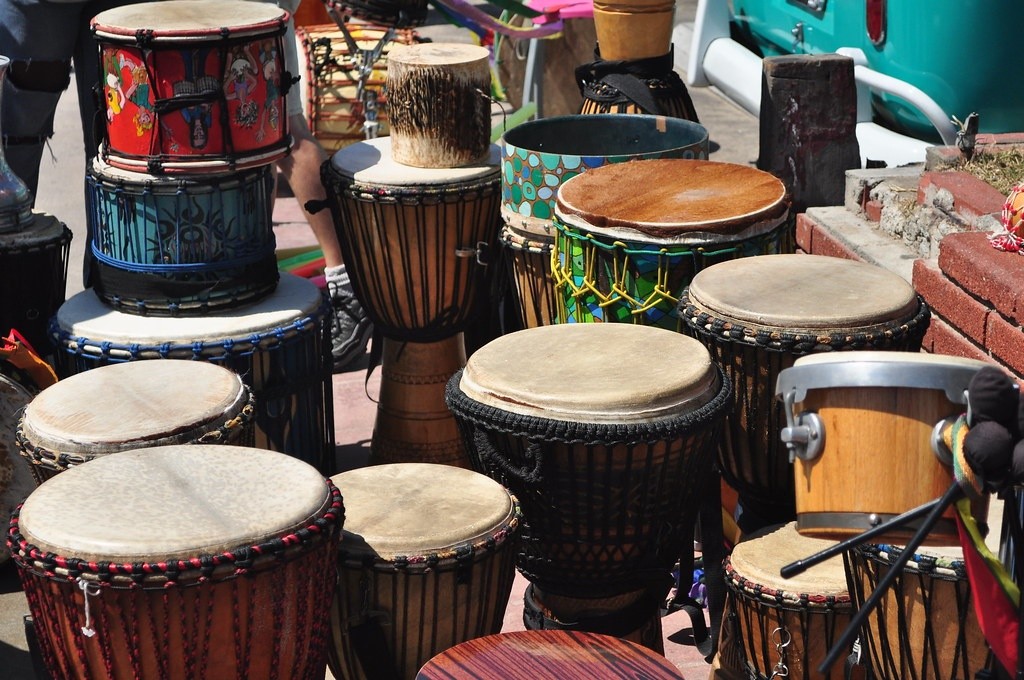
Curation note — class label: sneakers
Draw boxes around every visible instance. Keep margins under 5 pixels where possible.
[322,274,374,364]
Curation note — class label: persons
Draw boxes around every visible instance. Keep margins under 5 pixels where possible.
[260,0,373,373]
[1,1,150,288]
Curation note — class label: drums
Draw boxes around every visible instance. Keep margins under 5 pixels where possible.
[790,349,991,546]
[0,211,73,568]
[497,111,710,328]
[317,134,504,469]
[444,321,733,659]
[6,441,347,680]
[86,0,290,318]
[678,253,932,680]
[15,358,257,489]
[575,2,700,123]
[46,273,336,481]
[321,461,523,679]
[722,520,1010,679]
[288,20,421,137]
[550,159,792,335]
